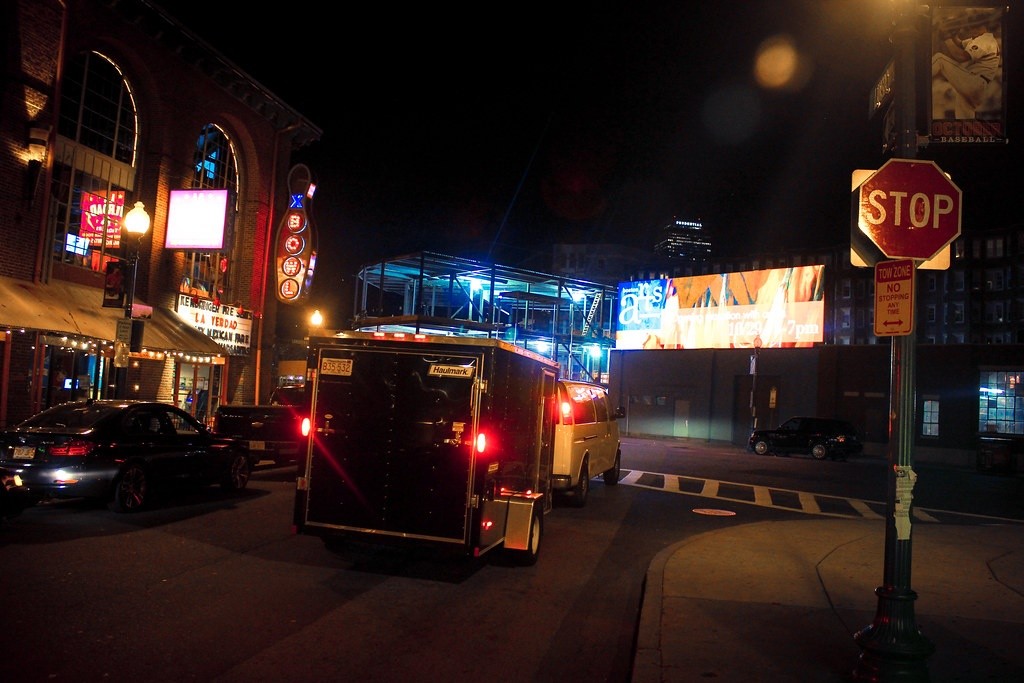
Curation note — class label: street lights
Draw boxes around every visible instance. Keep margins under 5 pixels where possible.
[114,202,152,399]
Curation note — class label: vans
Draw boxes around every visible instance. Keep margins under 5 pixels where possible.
[294,329,559,564]
[553,376,629,508]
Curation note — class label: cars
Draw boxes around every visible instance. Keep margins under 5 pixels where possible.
[0,396,253,543]
[748,416,866,459]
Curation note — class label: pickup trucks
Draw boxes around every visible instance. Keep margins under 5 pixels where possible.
[213,382,352,464]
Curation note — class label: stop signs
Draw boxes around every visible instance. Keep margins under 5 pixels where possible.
[859,161,963,261]
